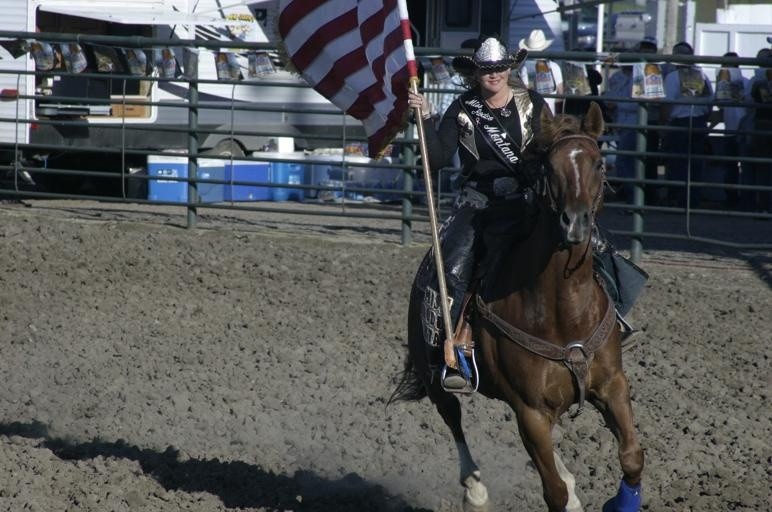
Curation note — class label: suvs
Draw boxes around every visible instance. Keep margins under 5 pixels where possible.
[561,10,657,55]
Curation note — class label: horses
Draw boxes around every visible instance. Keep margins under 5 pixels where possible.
[382,99,648,512]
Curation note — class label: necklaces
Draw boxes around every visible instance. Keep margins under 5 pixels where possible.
[484,90,512,118]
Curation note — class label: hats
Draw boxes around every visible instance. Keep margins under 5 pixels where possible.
[519,30,552,52]
[452,37,528,77]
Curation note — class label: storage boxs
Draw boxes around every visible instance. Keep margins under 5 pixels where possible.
[146,148,414,208]
[112,98,151,118]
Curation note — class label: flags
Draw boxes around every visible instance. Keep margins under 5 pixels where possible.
[278,0,421,158]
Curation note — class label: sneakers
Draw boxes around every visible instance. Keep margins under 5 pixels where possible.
[443,365,467,389]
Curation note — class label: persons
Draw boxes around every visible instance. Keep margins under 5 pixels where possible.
[518,32,772,198]
[406,31,636,387]
[421,55,472,198]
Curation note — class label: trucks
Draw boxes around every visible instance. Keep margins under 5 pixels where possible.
[0,2,464,199]
